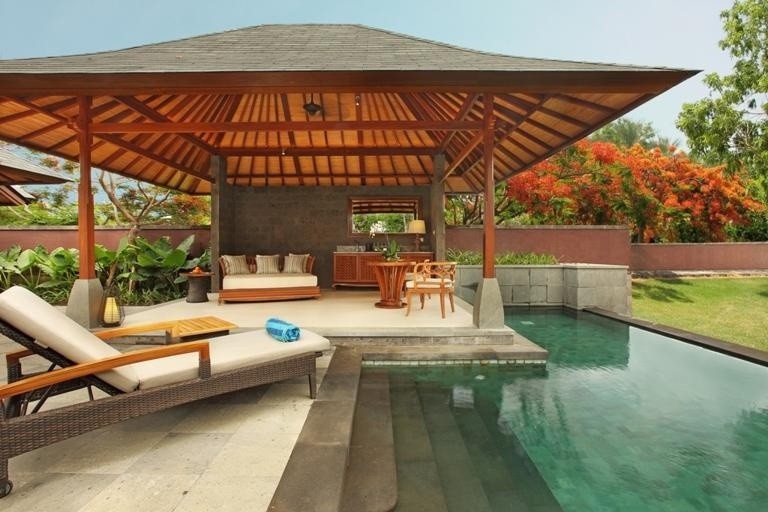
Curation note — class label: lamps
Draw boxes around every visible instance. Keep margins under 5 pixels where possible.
[96,282,125,327]
[303,93,322,116]
[407,219,427,251]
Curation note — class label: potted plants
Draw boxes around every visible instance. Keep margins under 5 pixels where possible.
[375,240,401,262]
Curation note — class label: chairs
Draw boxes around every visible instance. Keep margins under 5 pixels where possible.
[403,259,456,320]
[0,285,331,497]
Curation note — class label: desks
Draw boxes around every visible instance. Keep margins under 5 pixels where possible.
[367,261,416,309]
[179,271,216,303]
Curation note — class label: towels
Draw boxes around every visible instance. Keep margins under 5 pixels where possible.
[264,318,301,343]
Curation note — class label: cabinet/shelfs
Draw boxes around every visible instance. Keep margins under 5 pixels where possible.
[331,251,435,292]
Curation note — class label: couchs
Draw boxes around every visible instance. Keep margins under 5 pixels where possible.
[218,256,321,304]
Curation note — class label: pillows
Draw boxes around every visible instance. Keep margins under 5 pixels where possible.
[222,253,310,275]
